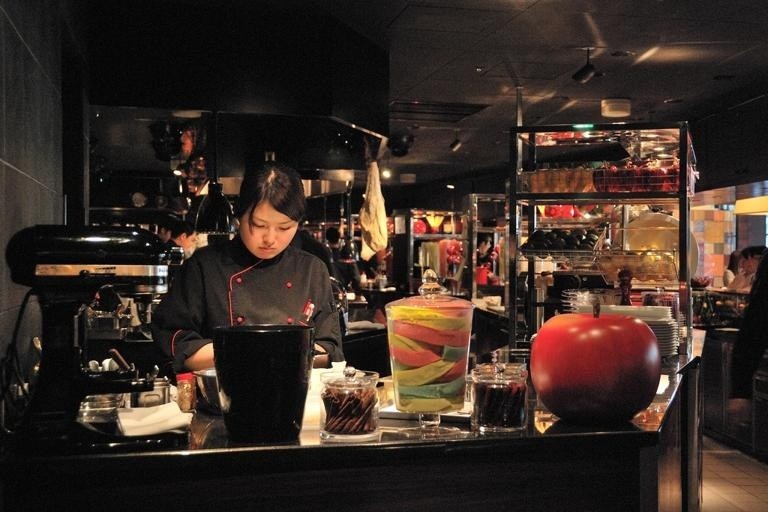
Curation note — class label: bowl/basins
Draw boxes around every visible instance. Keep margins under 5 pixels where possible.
[193,367,222,415]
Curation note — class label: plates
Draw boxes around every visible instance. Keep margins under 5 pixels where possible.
[640,319,680,356]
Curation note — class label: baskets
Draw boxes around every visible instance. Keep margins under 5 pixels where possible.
[524,166,696,196]
[517,228,606,262]
[596,249,677,285]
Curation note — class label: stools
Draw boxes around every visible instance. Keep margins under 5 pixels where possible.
[752,369,768,452]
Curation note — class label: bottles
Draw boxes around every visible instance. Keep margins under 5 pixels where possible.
[176,372,195,412]
[474,363,529,432]
[320,370,381,441]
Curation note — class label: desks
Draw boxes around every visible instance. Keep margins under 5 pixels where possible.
[713,326,740,430]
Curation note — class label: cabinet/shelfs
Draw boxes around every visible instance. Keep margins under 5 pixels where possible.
[472,195,625,326]
[512,127,696,354]
[409,210,508,311]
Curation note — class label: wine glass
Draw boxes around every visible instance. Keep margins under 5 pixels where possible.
[384,270,471,438]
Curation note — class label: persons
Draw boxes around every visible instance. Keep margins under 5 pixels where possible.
[723,245,768,424]
[150,160,345,374]
[155,216,366,302]
[477,234,500,276]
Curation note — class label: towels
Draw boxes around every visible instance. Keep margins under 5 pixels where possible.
[115,402,190,436]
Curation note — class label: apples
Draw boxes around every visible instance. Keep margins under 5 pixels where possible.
[529,302,661,423]
